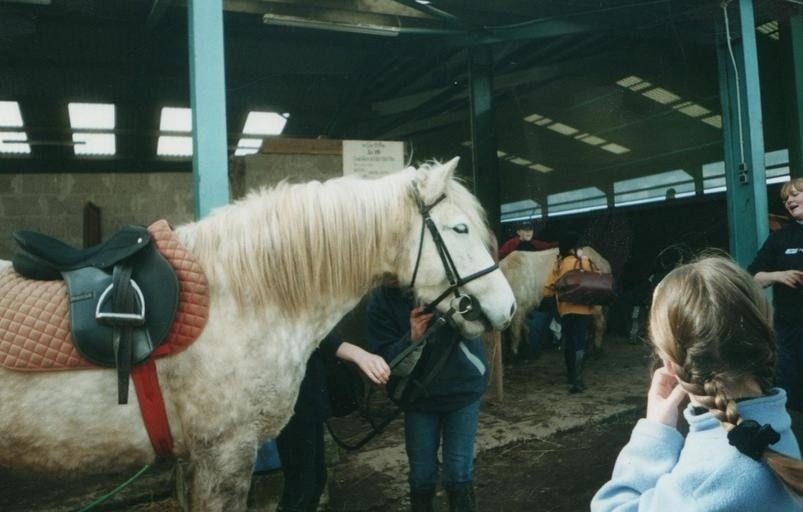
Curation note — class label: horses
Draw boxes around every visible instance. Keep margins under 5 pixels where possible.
[499,244,612,361]
[0,153,518,512]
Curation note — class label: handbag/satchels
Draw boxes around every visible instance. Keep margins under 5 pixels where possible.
[558,270,616,304]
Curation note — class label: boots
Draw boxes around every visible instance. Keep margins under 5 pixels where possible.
[407,489,437,512]
[563,348,586,393]
[442,480,476,512]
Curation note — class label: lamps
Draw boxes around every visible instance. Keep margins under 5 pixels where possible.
[262,13,400,38]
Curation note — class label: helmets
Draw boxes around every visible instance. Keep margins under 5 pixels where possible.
[559,231,586,255]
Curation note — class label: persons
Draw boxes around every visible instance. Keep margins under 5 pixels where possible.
[590,250,800,510]
[277,295,391,511]
[362,274,491,512]
[576,230,615,360]
[546,226,602,392]
[494,221,556,374]
[747,176,801,444]
[646,231,685,287]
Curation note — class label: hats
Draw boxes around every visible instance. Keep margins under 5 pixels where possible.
[519,221,535,231]
